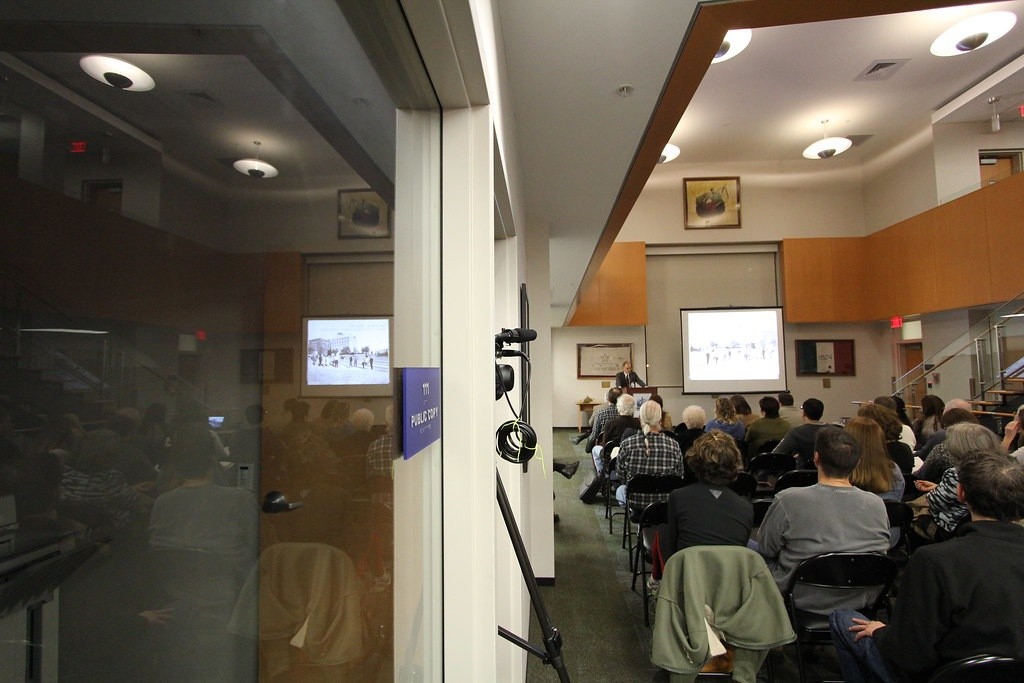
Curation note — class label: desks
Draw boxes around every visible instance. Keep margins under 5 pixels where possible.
[576,401,602,433]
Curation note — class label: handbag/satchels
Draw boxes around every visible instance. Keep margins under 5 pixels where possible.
[578,469,602,506]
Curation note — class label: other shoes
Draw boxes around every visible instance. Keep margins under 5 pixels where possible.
[647,576,660,598]
[569,436,581,445]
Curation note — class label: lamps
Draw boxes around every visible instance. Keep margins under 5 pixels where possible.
[232,142,278,178]
[802,119,852,159]
[929,11,1017,57]
[657,144,680,164]
[80,55,154,92]
[711,29,752,64]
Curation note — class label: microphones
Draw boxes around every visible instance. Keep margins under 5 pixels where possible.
[495,329,537,344]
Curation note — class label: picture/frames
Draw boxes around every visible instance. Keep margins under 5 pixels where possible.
[683,177,742,230]
[338,189,390,240]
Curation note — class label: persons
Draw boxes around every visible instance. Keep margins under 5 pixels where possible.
[745,423,891,664]
[647,428,754,598]
[569,387,1024,597]
[553,460,580,524]
[616,362,648,389]
[706,348,766,364]
[0,397,394,683]
[309,351,375,370]
[828,449,1024,683]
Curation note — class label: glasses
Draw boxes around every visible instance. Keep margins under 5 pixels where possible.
[800,405,804,409]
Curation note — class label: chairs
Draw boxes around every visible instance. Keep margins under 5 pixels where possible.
[593,424,1024,683]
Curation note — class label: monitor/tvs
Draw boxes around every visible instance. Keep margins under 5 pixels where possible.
[208,415,225,429]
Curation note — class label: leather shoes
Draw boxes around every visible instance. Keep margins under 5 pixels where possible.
[561,460,581,479]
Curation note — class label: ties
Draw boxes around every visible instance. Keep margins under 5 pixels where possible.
[625,375,630,385]
